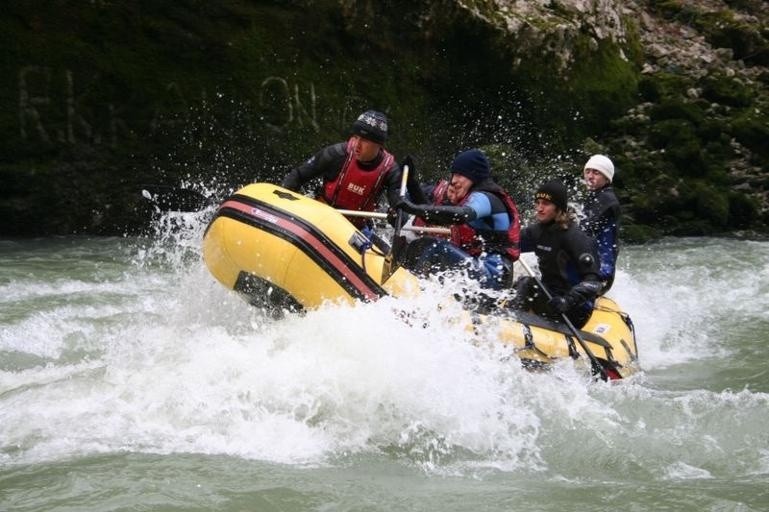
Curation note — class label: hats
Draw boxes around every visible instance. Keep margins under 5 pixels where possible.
[584,155,615,184]
[534,180,568,212]
[450,150,489,185]
[353,109,388,145]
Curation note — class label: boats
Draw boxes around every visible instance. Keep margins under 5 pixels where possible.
[204,181,638,377]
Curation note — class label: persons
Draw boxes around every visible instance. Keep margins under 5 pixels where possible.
[281,109,412,232]
[578,154,622,298]
[498,181,601,329]
[389,177,460,240]
[394,149,521,291]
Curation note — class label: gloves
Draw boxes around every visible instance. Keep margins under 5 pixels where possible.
[547,295,570,316]
[400,155,416,179]
[395,196,418,214]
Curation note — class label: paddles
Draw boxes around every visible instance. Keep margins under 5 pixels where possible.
[381,159,407,284]
[157,183,397,224]
[518,258,622,381]
